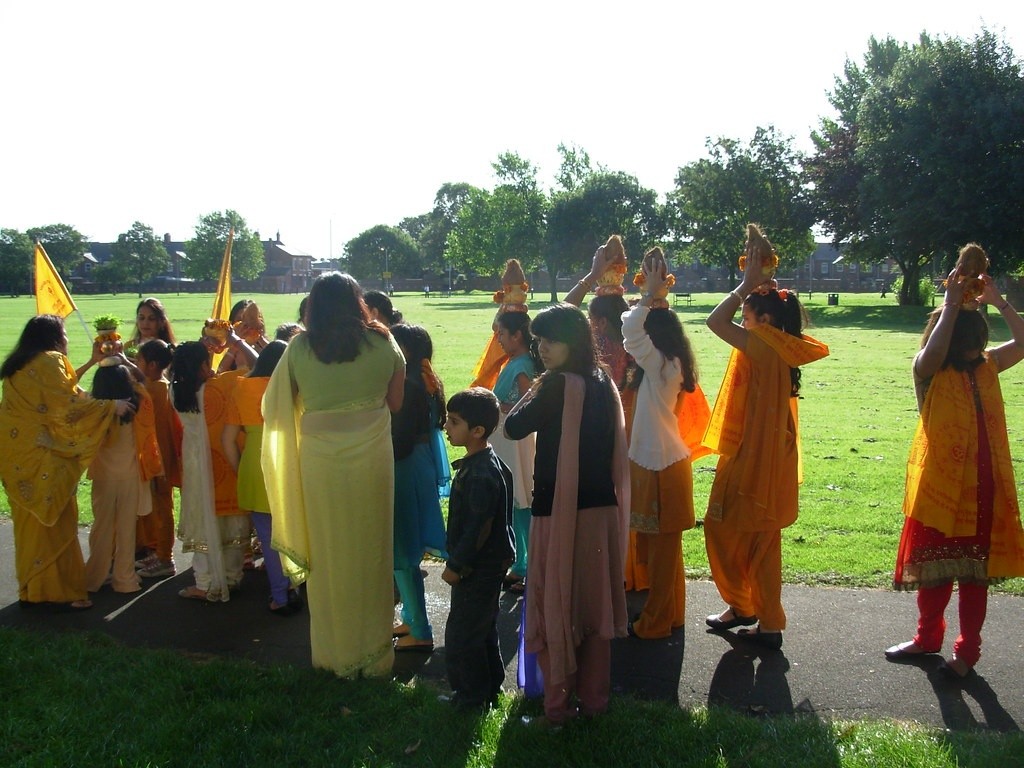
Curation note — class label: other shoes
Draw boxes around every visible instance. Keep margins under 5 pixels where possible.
[102,572,114,584]
[392,642,435,652]
[269,594,291,614]
[287,597,303,612]
[705,604,758,629]
[439,689,495,711]
[242,552,254,568]
[389,627,410,637]
[70,599,92,608]
[939,662,972,681]
[884,644,941,657]
[576,699,594,720]
[178,584,209,599]
[619,621,667,642]
[531,715,563,733]
[252,536,263,554]
[737,623,782,649]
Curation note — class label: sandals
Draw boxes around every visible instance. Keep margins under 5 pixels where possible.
[504,574,526,592]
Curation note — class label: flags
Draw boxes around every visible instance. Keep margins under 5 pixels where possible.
[211,245,231,373]
[34,247,75,321]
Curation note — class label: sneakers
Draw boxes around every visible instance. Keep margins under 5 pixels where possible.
[136,560,175,577]
[134,552,160,569]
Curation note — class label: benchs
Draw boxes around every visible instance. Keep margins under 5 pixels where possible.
[673,293,696,306]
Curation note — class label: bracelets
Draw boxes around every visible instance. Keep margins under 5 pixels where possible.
[578,280,591,292]
[731,291,743,306]
[1000,302,1008,310]
[945,302,961,309]
[233,339,245,347]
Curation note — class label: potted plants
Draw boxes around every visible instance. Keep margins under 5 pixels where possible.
[93,314,121,336]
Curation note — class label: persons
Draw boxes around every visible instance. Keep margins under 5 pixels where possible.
[75,340,153,592]
[261,270,406,680]
[621,258,697,639]
[502,305,628,726]
[438,386,517,711]
[387,323,452,652]
[886,263,1024,688]
[217,300,305,617]
[564,245,713,591]
[706,245,829,646]
[390,284,394,295]
[124,298,183,555]
[424,284,430,298]
[880,284,886,298]
[172,318,259,603]
[485,304,547,595]
[0,314,137,611]
[134,339,184,576]
[364,290,403,329]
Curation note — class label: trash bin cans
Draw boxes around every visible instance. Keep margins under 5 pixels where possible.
[828,294,838,305]
